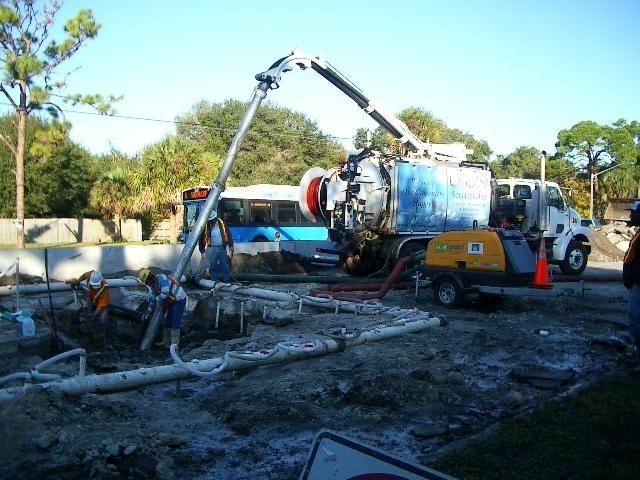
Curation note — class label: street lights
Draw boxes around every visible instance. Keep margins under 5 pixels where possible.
[589,161,634,221]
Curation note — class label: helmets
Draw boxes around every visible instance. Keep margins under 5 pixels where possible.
[89,270,103,286]
[138,268,151,285]
[208,209,218,220]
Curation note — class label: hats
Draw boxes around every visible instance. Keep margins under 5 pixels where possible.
[623,200,640,213]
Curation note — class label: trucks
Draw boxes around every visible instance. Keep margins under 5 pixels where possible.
[296,145,594,275]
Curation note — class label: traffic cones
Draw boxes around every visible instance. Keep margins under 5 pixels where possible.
[528,236,555,290]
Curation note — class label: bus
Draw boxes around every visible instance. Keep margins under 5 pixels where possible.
[181,184,333,246]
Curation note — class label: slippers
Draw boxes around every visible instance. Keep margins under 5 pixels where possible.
[164,328,181,349]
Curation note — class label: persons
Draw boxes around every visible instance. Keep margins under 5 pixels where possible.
[199,210,235,282]
[138,269,186,365]
[620,201,639,345]
[69,269,111,325]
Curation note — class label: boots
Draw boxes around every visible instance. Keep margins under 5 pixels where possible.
[155,327,171,346]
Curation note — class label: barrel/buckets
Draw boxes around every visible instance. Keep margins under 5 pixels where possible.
[21,312,36,336]
